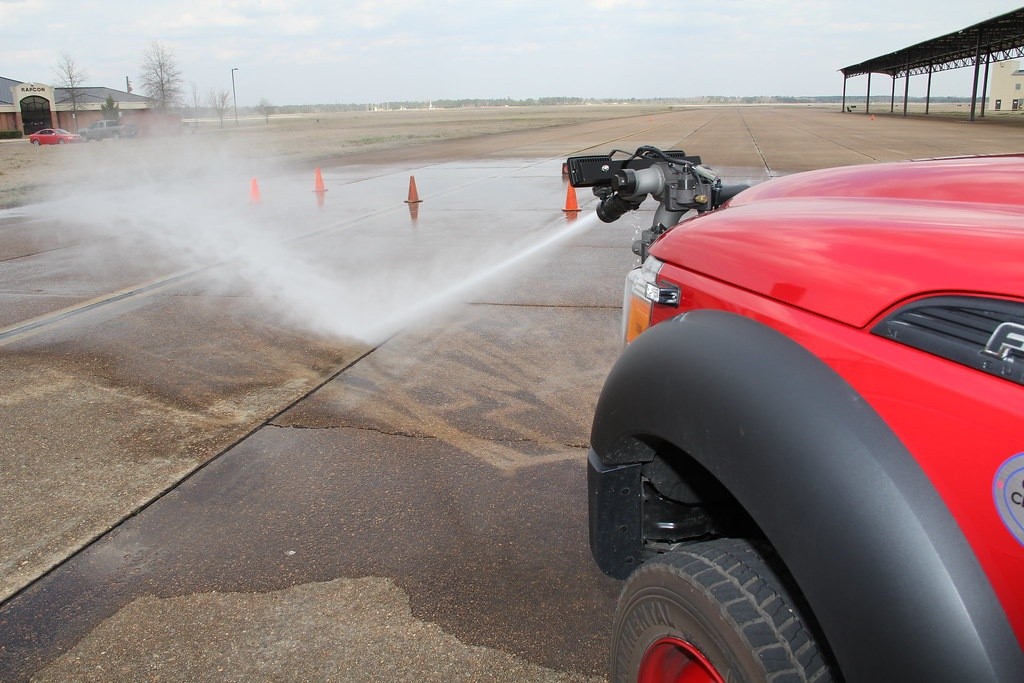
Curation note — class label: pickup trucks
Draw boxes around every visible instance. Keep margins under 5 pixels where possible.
[79,120,138,142]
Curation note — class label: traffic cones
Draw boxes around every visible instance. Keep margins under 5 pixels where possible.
[313,192,327,206]
[249,178,264,204]
[405,202,422,219]
[404,176,423,203]
[562,183,582,211]
[313,168,327,192]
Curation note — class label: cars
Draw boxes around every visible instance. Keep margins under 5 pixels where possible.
[29,128,82,146]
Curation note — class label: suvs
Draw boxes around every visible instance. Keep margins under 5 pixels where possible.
[566,147,1023,682]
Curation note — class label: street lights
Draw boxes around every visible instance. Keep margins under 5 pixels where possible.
[232,68,239,123]
